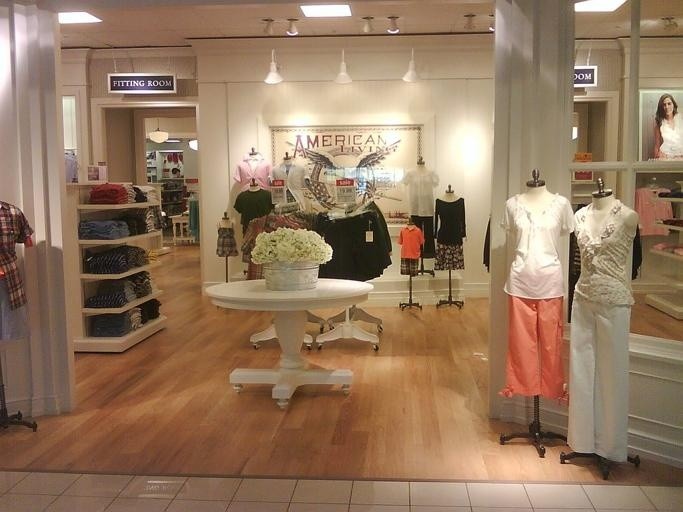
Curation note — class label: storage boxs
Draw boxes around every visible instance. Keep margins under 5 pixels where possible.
[574,153,592,180]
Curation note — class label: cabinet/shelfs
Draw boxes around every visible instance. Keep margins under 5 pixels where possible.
[146,150,198,216]
[645,175,683,318]
[63,182,170,355]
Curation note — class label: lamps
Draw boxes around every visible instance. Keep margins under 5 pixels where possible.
[487,12,495,32]
[360,16,375,34]
[263,48,284,85]
[401,49,421,82]
[262,18,276,35]
[284,18,301,37]
[385,16,401,36]
[148,118,168,144]
[336,47,352,85]
[461,14,475,31]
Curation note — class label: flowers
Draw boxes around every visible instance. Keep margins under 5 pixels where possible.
[248,227,337,268]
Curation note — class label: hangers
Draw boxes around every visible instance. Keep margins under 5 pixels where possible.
[645,175,683,190]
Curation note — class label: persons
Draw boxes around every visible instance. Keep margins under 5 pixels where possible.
[234,184,274,224]
[233,151,270,193]
[215,217,239,258]
[433,190,468,271]
[402,161,440,258]
[497,180,574,400]
[267,157,310,210]
[397,225,426,276]
[652,93,683,159]
[0,201,34,342]
[565,190,641,463]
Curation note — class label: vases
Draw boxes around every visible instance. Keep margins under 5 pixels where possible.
[261,259,320,291]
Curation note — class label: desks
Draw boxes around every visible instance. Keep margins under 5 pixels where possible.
[205,275,374,407]
[168,213,197,246]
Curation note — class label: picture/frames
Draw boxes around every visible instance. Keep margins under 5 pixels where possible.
[638,86,682,165]
[256,109,437,239]
